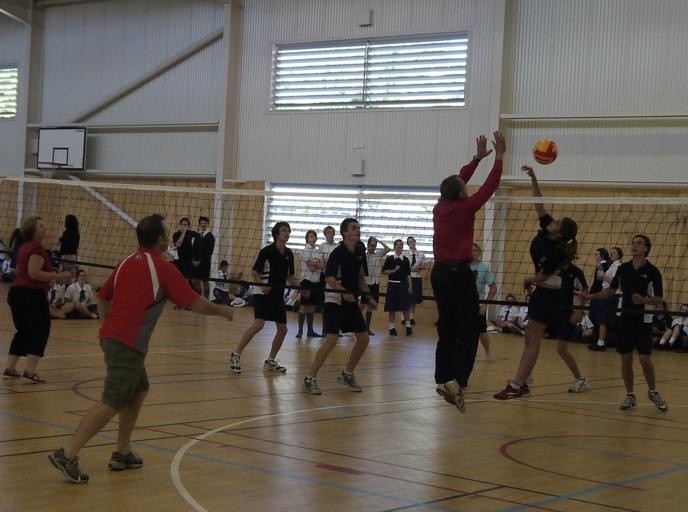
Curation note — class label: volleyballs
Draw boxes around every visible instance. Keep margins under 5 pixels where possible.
[532,138,558,164]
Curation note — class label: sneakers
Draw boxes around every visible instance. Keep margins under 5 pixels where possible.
[494,381,530,399]
[435,378,466,414]
[22,370,46,383]
[109,449,143,469]
[231,352,241,373]
[339,370,362,392]
[389,319,416,336]
[568,377,587,393]
[588,343,606,351]
[263,359,287,372]
[3,367,20,378]
[619,392,637,409]
[655,341,672,351]
[304,376,321,394]
[647,390,667,410]
[48,447,89,483]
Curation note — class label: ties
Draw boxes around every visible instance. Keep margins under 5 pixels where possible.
[80,290,85,302]
[50,291,55,304]
[505,311,509,321]
[412,254,416,267]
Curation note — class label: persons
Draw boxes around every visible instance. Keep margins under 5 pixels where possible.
[48,214,234,484]
[430,130,506,415]
[303,217,378,395]
[494,166,579,400]
[3,217,73,384]
[576,234,668,411]
[3,227,25,273]
[171,217,215,310]
[507,262,589,395]
[469,243,687,363]
[211,260,228,305]
[46,217,100,320]
[296,227,425,337]
[229,221,311,373]
